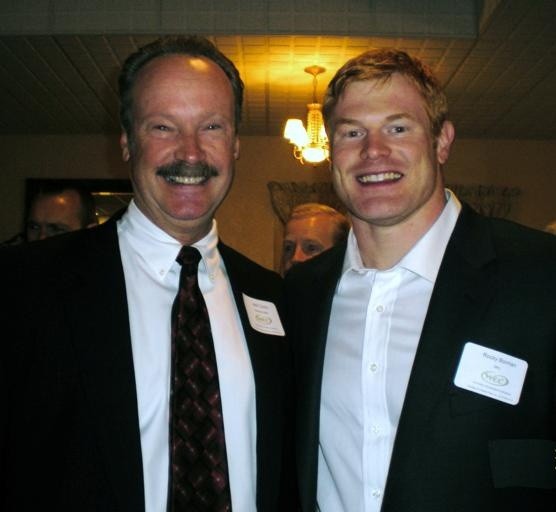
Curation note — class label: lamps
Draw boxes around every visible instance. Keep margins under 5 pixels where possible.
[282,65,330,166]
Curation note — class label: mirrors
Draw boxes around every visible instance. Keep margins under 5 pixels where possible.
[22,176,133,227]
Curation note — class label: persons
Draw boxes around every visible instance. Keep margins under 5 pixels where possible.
[0,34,288,512]
[283,50,554,512]
[283,202,350,275]
[24,181,98,242]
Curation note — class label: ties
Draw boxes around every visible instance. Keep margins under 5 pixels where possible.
[166,245,232,512]
